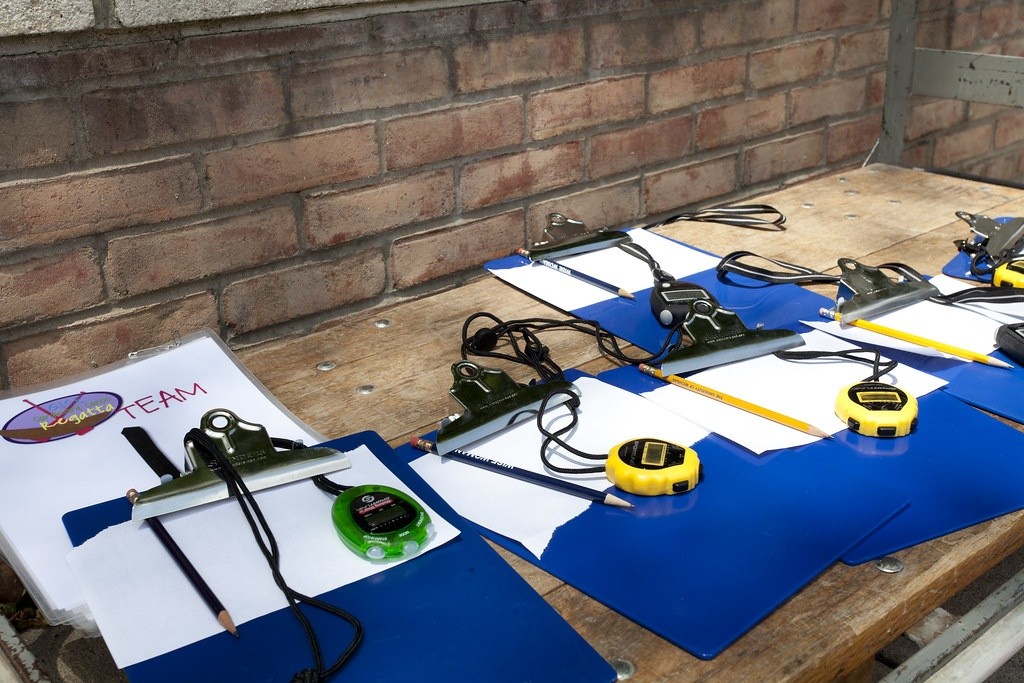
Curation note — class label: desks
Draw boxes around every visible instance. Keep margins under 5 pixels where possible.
[0,163,1024,681]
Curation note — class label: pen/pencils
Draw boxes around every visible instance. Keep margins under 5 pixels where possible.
[517,247,637,301]
[408,435,635,510]
[127,488,241,638]
[637,362,836,442]
[818,307,1017,369]
